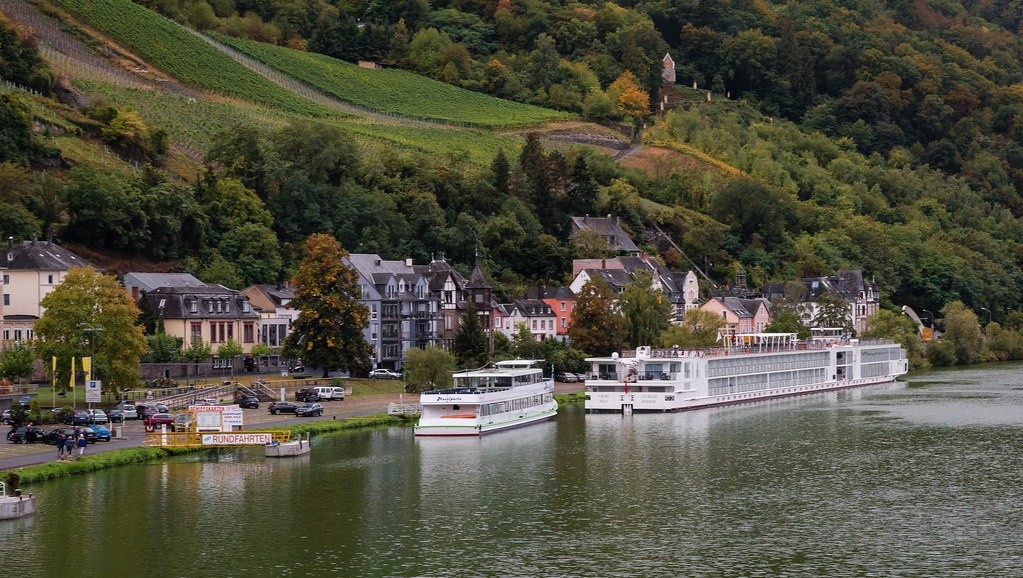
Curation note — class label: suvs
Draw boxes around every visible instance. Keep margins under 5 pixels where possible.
[295,388,319,402]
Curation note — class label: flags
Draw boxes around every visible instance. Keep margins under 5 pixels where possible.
[715,332,722,342]
[729,334,734,341]
[624,378,629,394]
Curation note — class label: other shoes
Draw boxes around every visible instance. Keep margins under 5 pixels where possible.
[61,455,64,460]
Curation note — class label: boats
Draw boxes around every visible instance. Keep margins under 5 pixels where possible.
[583,328,910,411]
[412,357,559,437]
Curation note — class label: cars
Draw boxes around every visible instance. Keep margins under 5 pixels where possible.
[62,410,90,426]
[267,402,299,415]
[369,369,403,380]
[290,366,305,373]
[85,409,108,424]
[44,424,112,445]
[295,403,324,417]
[556,372,577,384]
[575,373,586,381]
[20,397,30,405]
[6,426,48,445]
[51,407,71,420]
[1,409,23,425]
[194,399,220,407]
[170,415,193,432]
[234,396,259,409]
[105,402,170,422]
[144,414,176,432]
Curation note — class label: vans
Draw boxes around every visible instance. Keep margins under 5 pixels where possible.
[330,387,345,401]
[313,387,332,402]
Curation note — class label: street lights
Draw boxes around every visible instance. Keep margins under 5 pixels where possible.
[982,308,991,328]
[923,310,934,329]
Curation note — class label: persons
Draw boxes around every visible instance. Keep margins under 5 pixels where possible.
[56,435,65,457]
[64,436,75,454]
[6,471,19,497]
[77,434,87,455]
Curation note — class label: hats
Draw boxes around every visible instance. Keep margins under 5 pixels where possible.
[80,434,83,437]
[68,436,72,439]
